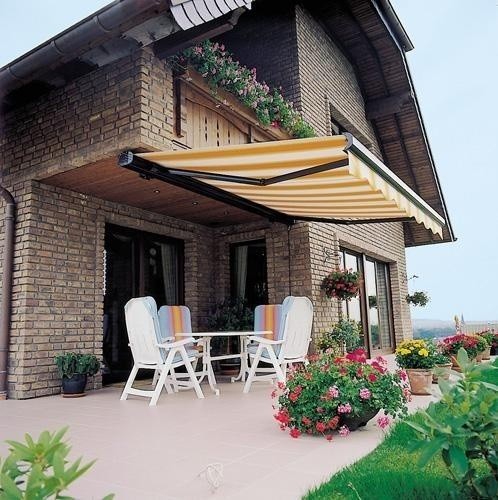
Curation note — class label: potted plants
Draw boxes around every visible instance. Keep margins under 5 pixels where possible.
[202,296,254,376]
[54,351,100,394]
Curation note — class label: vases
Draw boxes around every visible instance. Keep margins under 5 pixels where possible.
[431,362,453,384]
[406,368,433,395]
[335,407,379,432]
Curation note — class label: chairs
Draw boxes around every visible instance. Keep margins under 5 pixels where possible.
[243,296,314,392]
[158,306,216,392]
[120,296,205,405]
[231,303,283,383]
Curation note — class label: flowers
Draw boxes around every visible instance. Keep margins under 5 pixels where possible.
[170,39,317,139]
[320,266,364,303]
[395,330,498,370]
[271,350,413,442]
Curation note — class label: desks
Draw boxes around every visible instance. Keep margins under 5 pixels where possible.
[175,330,273,396]
